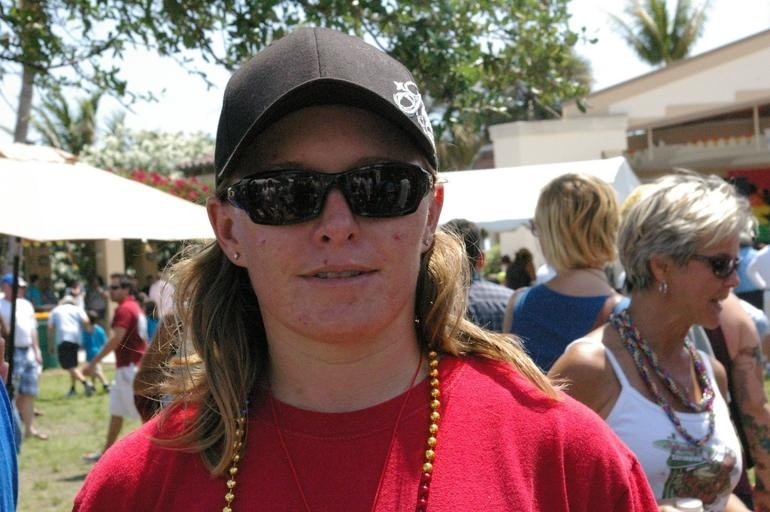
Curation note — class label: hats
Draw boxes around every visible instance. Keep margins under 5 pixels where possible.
[214,24,438,188]
[0,272,29,288]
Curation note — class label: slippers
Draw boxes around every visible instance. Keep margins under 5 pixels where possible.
[26,432,50,441]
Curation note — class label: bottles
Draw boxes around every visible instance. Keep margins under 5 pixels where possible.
[676,497,705,512]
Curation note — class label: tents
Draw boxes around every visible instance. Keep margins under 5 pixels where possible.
[2,142,217,242]
[428,156,643,246]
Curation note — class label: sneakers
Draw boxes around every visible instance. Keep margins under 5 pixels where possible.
[81,452,101,461]
[64,384,111,398]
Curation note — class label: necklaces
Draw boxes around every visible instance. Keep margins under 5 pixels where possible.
[222,341,439,511]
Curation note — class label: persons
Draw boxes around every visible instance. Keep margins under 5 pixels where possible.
[0,265,186,459]
[425,175,770,512]
[73,26,659,511]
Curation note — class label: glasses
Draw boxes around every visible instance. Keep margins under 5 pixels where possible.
[684,254,741,279]
[221,160,431,227]
[109,285,119,290]
